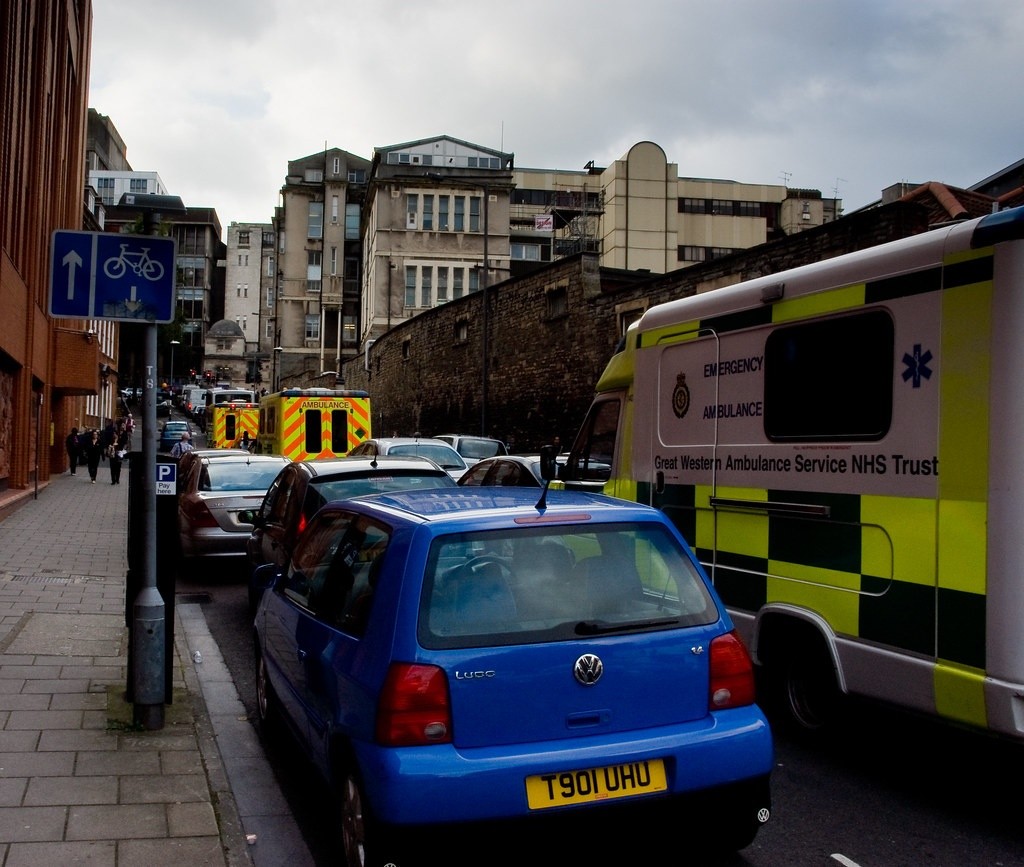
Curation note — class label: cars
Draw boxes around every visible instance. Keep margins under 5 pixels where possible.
[172,448,252,484]
[347,432,613,493]
[238,452,486,623]
[120,383,206,423]
[175,454,294,580]
[254,484,775,867]
[157,421,193,451]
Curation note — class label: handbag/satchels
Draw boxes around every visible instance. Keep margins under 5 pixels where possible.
[104,446,115,458]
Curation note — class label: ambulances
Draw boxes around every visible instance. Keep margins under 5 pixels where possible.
[205,401,259,451]
[540,204,1023,767]
[254,385,372,464]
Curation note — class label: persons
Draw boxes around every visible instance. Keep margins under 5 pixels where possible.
[170,432,195,459]
[124,413,137,450]
[65,420,129,485]
[240,431,251,449]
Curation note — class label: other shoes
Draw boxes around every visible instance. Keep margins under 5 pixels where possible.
[111,480,116,484]
[91,479,96,484]
[116,481,119,484]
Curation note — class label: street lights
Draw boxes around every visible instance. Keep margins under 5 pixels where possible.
[169,341,181,386]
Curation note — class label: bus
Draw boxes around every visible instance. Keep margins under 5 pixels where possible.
[200,385,256,405]
